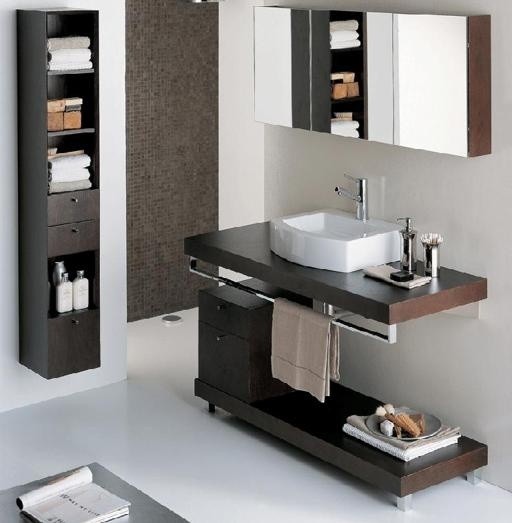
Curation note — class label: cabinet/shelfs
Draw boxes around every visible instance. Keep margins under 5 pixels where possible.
[193,277,490,512]
[291,9,369,140]
[15,4,101,381]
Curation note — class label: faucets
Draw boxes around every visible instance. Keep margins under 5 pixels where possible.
[334,173,368,220]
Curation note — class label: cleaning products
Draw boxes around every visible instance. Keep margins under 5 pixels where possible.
[397,216,418,271]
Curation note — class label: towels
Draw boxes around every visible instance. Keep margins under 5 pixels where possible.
[331,41,360,50]
[51,168,89,181]
[48,62,92,70]
[331,119,358,130]
[331,31,358,40]
[329,19,357,31]
[48,49,93,61]
[269,299,340,404]
[331,130,357,137]
[50,156,91,168]
[48,183,91,192]
[344,413,462,461]
[46,37,90,48]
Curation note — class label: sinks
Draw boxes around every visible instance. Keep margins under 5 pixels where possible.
[270,206,404,273]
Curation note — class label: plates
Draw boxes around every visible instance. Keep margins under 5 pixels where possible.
[365,407,444,442]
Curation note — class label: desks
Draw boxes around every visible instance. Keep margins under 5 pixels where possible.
[0,460,188,523]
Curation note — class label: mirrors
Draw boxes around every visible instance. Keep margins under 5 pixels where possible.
[252,4,490,160]
